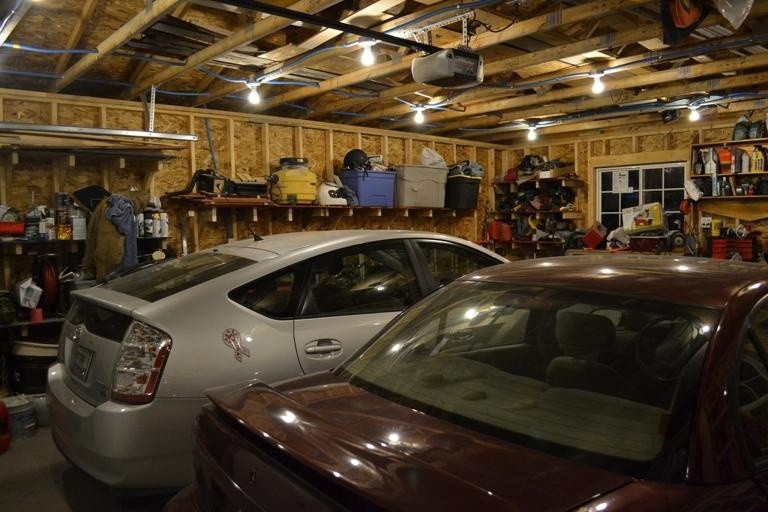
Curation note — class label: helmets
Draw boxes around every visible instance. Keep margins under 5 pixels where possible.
[344,149,367,170]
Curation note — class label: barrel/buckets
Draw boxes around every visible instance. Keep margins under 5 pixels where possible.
[6,396,39,442]
[268,156,317,204]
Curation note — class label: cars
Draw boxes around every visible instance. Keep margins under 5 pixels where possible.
[163,248,768,512]
[43,227,512,494]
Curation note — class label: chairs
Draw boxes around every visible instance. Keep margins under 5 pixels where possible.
[548,311,639,401]
[312,250,354,312]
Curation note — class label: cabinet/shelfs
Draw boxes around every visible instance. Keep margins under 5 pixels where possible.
[3,231,172,396]
[689,138,768,200]
[492,176,584,250]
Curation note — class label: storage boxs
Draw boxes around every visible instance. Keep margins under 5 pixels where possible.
[342,165,482,209]
